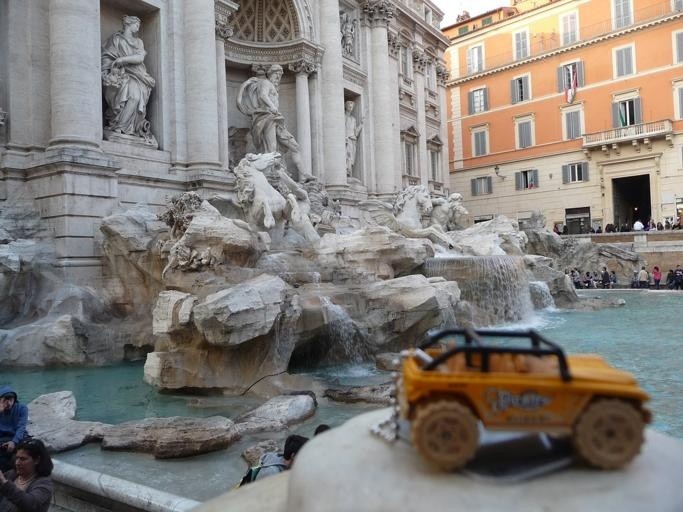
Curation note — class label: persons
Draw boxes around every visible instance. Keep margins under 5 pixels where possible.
[250,64,318,184]
[237,433,309,488]
[567,266,616,289]
[577,214,681,234]
[0,438,54,511]
[102,16,155,139]
[429,193,469,233]
[344,100,365,184]
[554,224,562,235]
[0,386,32,474]
[630,264,683,290]
[313,423,328,437]
[562,226,568,235]
[340,17,356,56]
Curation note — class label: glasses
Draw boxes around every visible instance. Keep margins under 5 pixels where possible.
[22,437,37,446]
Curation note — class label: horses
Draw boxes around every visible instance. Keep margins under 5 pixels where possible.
[232,151,301,249]
[393,185,465,253]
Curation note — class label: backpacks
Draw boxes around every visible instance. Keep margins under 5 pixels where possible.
[233,453,286,489]
